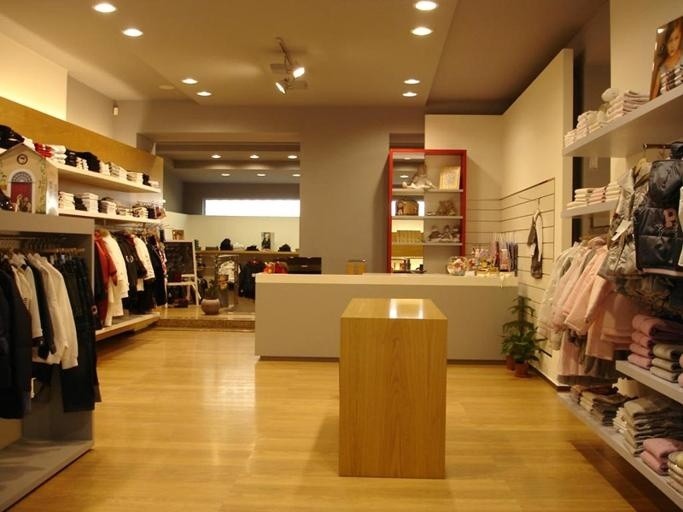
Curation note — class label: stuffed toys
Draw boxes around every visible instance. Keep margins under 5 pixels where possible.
[422,198,460,243]
[401,164,436,190]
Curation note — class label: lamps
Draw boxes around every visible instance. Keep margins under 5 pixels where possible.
[271,36,310,96]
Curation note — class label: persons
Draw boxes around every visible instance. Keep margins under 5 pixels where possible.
[648,18,682,101]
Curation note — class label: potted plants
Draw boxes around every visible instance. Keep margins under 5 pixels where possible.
[501,295,539,378]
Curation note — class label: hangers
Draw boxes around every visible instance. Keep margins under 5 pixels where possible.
[579,228,616,243]
[95,226,152,237]
[635,143,648,175]
[1,238,83,255]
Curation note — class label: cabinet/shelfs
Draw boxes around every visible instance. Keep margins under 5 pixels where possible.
[388,147,466,273]
[197,252,299,308]
[548,83,683,512]
[0,140,165,340]
[164,239,199,306]
[1,211,99,511]
